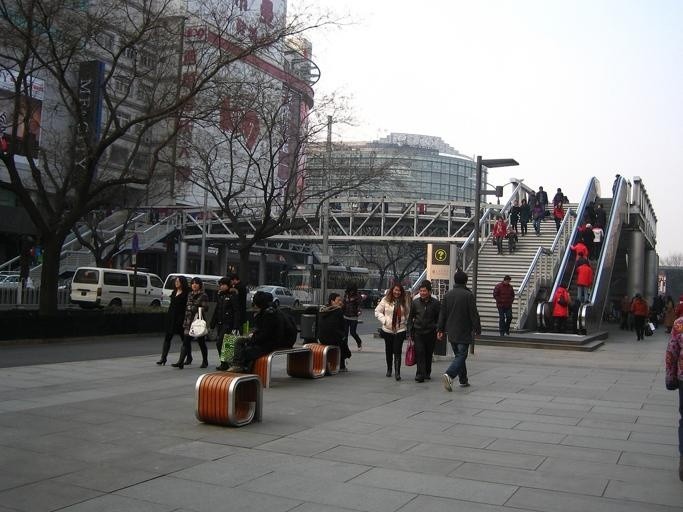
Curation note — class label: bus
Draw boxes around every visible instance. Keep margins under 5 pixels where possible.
[280,263,372,311]
[368,269,421,292]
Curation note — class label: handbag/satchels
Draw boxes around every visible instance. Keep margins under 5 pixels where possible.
[644,322,655,336]
[406,337,419,366]
[189,315,209,338]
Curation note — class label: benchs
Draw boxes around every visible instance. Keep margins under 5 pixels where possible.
[246,341,344,389]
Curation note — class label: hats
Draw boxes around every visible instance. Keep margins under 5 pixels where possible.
[254,290,273,308]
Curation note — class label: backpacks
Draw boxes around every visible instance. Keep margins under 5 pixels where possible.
[272,306,298,347]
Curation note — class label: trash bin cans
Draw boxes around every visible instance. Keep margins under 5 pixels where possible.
[300,304,325,344]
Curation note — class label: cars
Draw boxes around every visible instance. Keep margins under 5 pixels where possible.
[356,290,385,308]
[0,274,35,289]
[247,283,300,309]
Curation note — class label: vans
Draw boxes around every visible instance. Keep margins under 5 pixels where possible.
[68,265,165,311]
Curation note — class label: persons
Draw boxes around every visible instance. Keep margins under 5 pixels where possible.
[612,174,620,196]
[27,240,41,267]
[493,186,605,333]
[154,207,159,223]
[492,274,515,337]
[435,270,482,391]
[406,283,441,382]
[149,208,153,224]
[616,294,676,341]
[662,298,683,482]
[449,202,457,216]
[465,202,471,218]
[674,294,682,324]
[328,194,388,213]
[373,281,409,381]
[158,266,363,376]
[418,200,427,214]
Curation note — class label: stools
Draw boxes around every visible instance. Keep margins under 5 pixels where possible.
[191,370,263,429]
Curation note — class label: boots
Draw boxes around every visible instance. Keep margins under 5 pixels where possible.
[200,347,209,368]
[386,352,393,378]
[155,352,168,369]
[184,350,193,365]
[394,353,401,381]
[171,352,187,369]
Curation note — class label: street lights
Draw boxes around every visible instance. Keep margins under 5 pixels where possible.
[468,176,525,219]
[466,154,521,355]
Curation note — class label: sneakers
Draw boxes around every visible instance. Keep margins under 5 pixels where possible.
[215,359,230,372]
[414,370,433,381]
[499,327,512,337]
[441,372,455,391]
[358,340,363,352]
[458,380,472,387]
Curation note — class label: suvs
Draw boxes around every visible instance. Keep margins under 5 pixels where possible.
[159,271,226,310]
